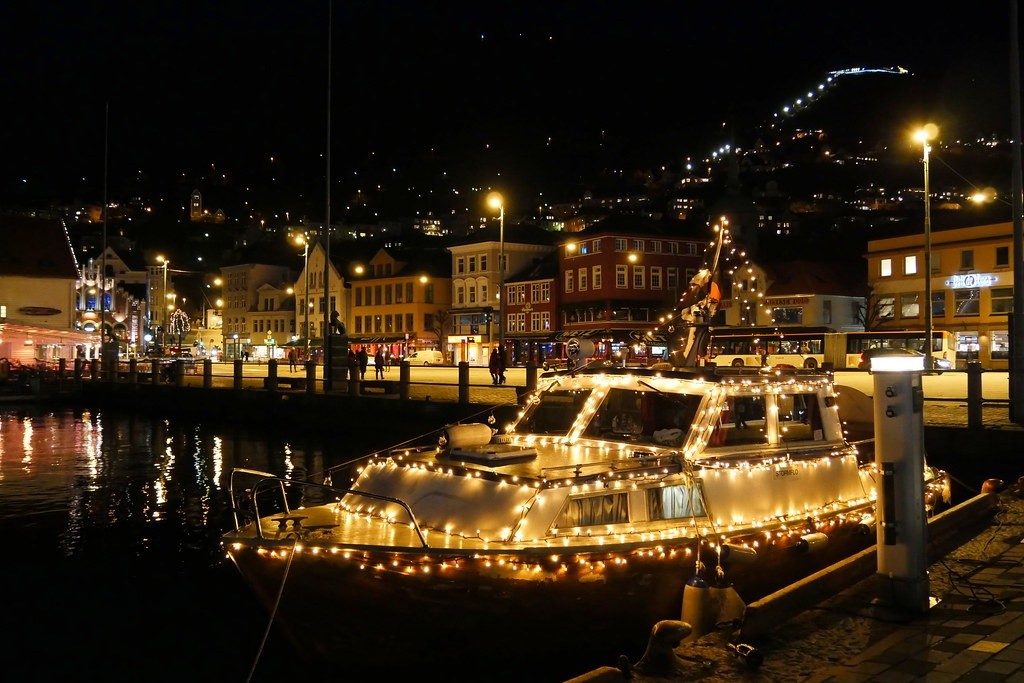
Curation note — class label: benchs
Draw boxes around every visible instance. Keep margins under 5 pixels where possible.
[360,381,401,394]
[264,377,308,391]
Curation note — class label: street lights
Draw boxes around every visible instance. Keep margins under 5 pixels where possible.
[155,256,167,348]
[295,237,310,368]
[912,129,936,377]
[489,196,505,346]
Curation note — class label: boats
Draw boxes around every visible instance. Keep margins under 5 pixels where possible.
[221,369,950,683]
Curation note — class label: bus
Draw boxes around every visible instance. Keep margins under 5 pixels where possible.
[707,326,957,373]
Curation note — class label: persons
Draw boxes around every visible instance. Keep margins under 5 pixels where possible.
[567,351,576,370]
[722,339,876,354]
[237,344,510,386]
[662,349,668,362]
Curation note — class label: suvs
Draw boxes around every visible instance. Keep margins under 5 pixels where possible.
[858,345,951,371]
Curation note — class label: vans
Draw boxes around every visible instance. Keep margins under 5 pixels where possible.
[403,350,443,367]
